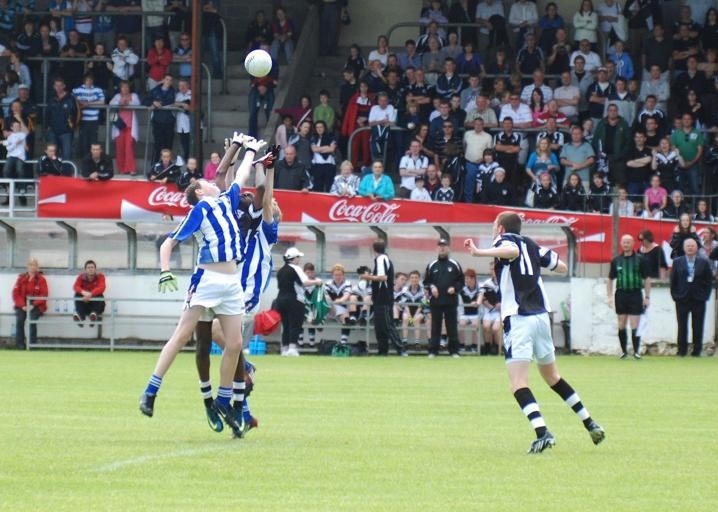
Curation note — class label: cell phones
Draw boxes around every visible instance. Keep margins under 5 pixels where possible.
[370,66,376,71]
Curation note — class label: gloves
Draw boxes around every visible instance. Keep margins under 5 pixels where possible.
[231,131,254,144]
[252,152,273,167]
[224,138,242,165]
[266,144,283,169]
[245,139,266,152]
[157,271,179,294]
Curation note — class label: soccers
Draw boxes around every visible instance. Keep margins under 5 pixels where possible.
[243,48,272,78]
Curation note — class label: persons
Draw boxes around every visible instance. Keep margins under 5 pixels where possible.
[0,0,718,224]
[72,260,107,326]
[462,211,606,453]
[138,132,282,439]
[607,213,718,358]
[271,239,504,356]
[12,258,48,351]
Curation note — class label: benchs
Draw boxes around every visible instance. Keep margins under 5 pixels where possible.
[268,322,484,346]
[0,311,194,353]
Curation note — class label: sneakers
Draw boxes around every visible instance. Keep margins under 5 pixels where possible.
[632,353,641,360]
[242,416,260,437]
[349,314,357,327]
[72,314,85,329]
[138,393,159,418]
[212,398,240,431]
[247,363,256,395]
[88,311,97,329]
[282,348,299,357]
[587,423,606,449]
[523,430,556,454]
[204,397,223,433]
[617,352,628,360]
[358,317,366,330]
[231,405,246,437]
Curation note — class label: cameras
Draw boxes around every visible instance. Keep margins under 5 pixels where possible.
[357,266,371,274]
[558,46,565,52]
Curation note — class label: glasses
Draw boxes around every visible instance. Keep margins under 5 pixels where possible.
[638,238,646,242]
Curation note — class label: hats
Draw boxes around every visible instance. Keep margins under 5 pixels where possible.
[283,246,305,259]
[596,67,609,74]
[17,83,31,90]
[437,238,451,247]
